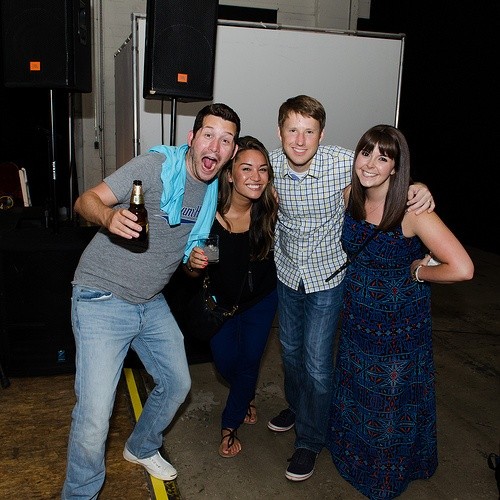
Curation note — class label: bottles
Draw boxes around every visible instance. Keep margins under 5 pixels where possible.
[128,180,150,253]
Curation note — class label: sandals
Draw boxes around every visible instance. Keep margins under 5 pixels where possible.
[244,404,258,424]
[218,428,242,458]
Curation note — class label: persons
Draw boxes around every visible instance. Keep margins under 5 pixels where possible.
[60,103,281,500]
[184,136,280,458]
[267,94,436,482]
[272,125,474,500]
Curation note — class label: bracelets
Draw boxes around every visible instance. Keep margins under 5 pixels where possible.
[412,264,424,284]
[185,263,195,274]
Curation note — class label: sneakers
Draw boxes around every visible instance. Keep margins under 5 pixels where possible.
[122,442,177,480]
[285,448,319,481]
[267,408,296,431]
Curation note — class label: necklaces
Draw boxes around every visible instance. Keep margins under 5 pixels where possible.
[364,200,385,217]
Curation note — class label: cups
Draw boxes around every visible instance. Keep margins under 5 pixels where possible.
[197,233,220,263]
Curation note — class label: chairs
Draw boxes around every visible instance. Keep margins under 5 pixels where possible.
[0,161,24,201]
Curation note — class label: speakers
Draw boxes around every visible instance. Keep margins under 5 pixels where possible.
[143,0,219,101]
[0,0,92,92]
